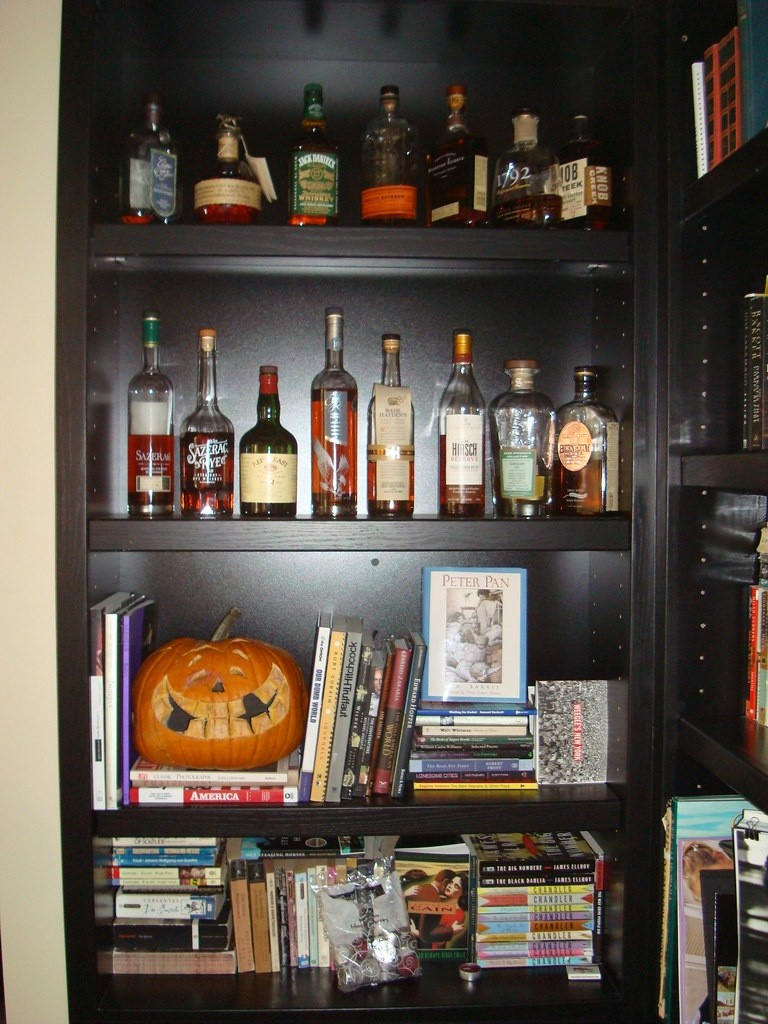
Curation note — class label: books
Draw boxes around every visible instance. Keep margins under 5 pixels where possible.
[656,794,768,1024]
[691,0,768,180]
[421,566,529,704]
[89,592,539,810]
[745,585,768,726]
[111,831,604,974]
[743,293,768,450]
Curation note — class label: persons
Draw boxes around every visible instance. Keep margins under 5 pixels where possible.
[682,841,735,903]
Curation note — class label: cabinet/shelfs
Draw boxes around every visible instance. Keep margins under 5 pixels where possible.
[57,0,768,1024]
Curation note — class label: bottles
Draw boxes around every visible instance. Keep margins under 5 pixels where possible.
[194,115,262,223]
[426,84,487,225]
[492,107,624,230]
[116,92,177,224]
[283,83,341,226]
[180,328,234,521]
[360,85,421,223]
[368,334,414,520]
[240,366,298,517]
[311,308,357,521]
[556,365,621,516]
[127,311,173,521]
[438,329,484,521]
[488,359,557,518]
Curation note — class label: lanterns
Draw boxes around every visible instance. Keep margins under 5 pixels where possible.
[131,636,310,770]
